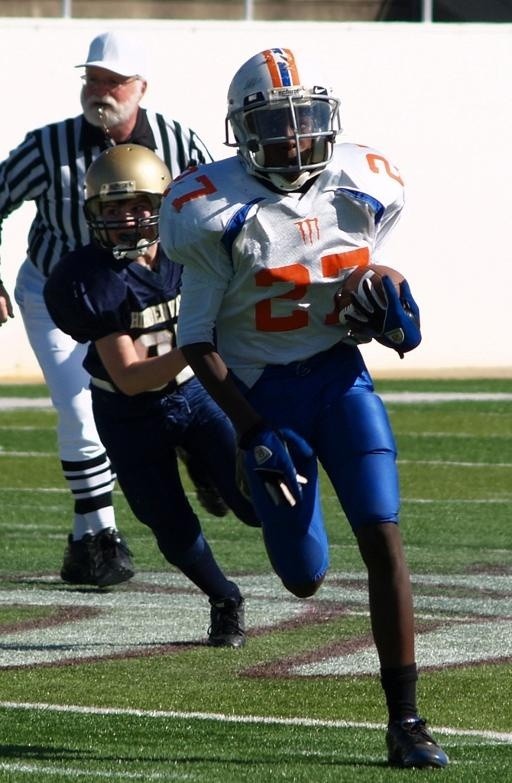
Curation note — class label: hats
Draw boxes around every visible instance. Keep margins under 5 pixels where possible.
[74,32,151,77]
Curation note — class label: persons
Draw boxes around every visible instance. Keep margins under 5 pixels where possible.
[0,28,212,591]
[158,44,456,770]
[40,139,264,652]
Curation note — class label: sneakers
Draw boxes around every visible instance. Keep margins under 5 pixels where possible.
[176,446,228,517]
[206,580,245,647]
[61,526,135,588]
[385,714,447,768]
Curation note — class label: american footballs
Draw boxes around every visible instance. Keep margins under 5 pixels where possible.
[335,263,406,330]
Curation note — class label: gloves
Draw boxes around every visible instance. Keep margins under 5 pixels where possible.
[344,276,421,359]
[239,428,317,508]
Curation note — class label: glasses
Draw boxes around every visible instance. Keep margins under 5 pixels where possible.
[80,74,139,85]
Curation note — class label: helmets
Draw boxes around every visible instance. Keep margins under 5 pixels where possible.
[223,48,342,193]
[82,143,173,260]
